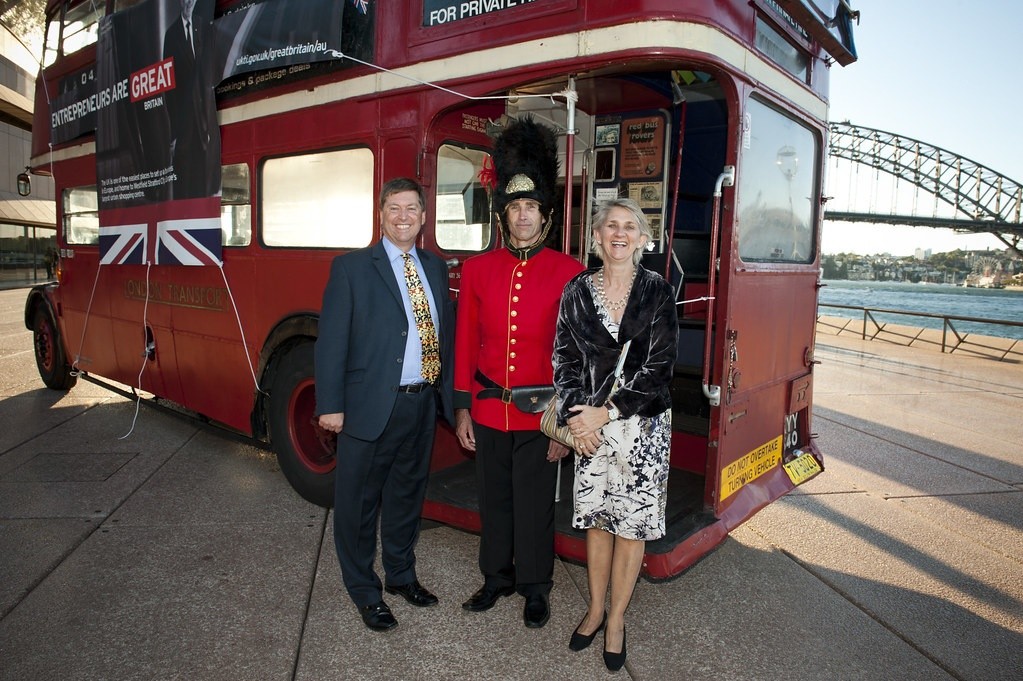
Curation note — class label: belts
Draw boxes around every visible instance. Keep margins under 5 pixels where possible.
[474,371,514,404]
[399,383,429,394]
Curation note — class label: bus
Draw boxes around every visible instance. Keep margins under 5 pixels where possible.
[18,1,861,585]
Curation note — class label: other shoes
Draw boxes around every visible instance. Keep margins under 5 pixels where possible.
[569,610,607,650]
[604,622,626,671]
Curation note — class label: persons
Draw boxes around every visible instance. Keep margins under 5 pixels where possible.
[45,247,60,279]
[552,198,679,672]
[314,178,458,631]
[454,199,586,628]
[164,0,221,200]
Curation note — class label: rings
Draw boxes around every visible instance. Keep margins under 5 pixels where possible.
[579,444,584,448]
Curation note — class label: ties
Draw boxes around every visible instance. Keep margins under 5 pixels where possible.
[401,253,441,385]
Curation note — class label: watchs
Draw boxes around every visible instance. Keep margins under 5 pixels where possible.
[605,402,619,420]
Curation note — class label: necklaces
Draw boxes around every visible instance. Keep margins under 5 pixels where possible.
[598,267,637,311]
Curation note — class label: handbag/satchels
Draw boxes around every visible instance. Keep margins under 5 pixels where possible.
[539,395,602,449]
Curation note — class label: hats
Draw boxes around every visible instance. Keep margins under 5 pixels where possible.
[493,115,558,213]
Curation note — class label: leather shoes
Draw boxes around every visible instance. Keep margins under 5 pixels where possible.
[462,582,515,611]
[385,580,438,607]
[358,600,399,631]
[523,594,550,628]
